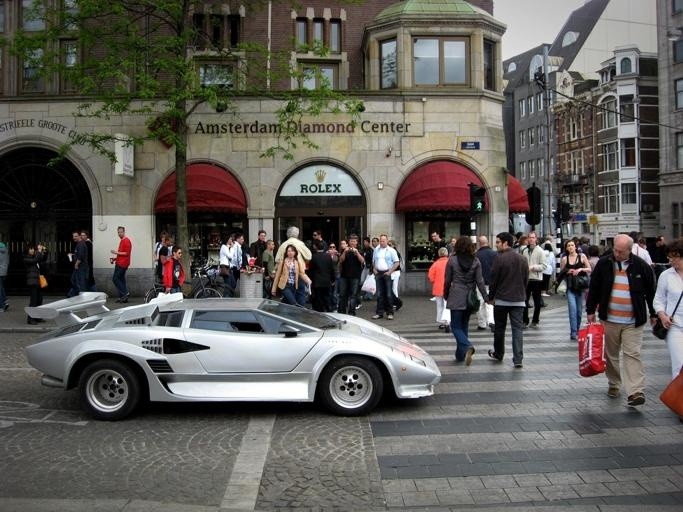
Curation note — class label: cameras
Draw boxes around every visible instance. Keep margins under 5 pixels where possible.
[349,248,353,252]
[40,246,47,252]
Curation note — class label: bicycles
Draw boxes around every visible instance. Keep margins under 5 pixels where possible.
[143,257,237,304]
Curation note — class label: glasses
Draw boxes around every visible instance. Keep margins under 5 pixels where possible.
[329,247,335,248]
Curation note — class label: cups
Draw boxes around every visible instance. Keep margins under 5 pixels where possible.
[248,258,255,266]
[110,258,113,264]
[67,254,72,262]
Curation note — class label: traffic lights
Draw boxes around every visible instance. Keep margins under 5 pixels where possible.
[562,202,572,222]
[471,184,485,216]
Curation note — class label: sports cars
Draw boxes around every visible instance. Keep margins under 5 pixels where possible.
[23,287,442,420]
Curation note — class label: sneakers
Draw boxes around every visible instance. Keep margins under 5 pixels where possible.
[514,364,522,367]
[607,388,619,397]
[438,322,539,332]
[627,393,645,405]
[488,350,503,360]
[465,349,473,365]
[372,315,383,318]
[388,314,394,320]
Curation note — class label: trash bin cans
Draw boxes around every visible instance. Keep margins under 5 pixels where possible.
[240,269,263,299]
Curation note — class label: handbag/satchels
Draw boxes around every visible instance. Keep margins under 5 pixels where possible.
[219,268,230,276]
[263,275,280,297]
[468,289,480,312]
[659,367,683,418]
[652,319,672,339]
[574,274,588,290]
[39,275,48,289]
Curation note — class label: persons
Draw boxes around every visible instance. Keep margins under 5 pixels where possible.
[66,230,97,298]
[219,230,244,297]
[585,231,682,406]
[109,226,132,303]
[428,230,607,368]
[237,227,404,320]
[21,244,49,325]
[1,240,9,312]
[153,231,186,294]
